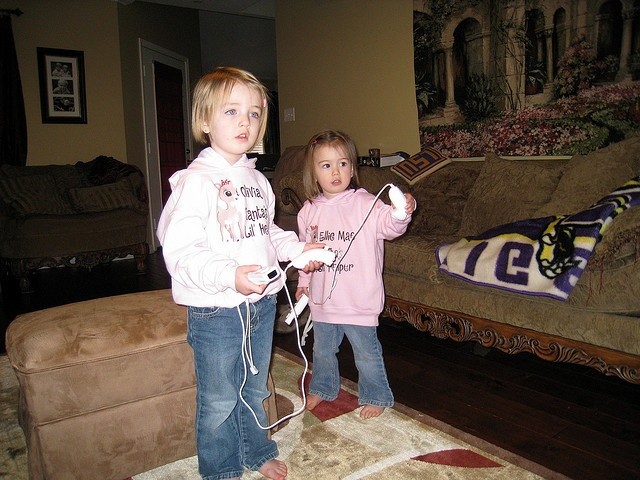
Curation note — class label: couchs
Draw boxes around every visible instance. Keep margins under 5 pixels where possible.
[0,155,151,295]
[272,147,639,383]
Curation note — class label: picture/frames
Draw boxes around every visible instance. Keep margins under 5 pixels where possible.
[36,47,87,125]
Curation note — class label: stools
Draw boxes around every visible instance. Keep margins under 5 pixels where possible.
[5,286,280,480]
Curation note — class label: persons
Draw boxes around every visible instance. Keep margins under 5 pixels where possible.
[52,62,63,76]
[61,64,71,77]
[292,128,417,420]
[155,63,333,480]
[53,78,70,94]
[54,100,71,111]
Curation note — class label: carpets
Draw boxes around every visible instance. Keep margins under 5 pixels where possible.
[0,344,575,479]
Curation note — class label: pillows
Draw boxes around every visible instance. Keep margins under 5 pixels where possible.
[456,153,567,242]
[566,205,639,316]
[68,178,136,214]
[531,136,639,217]
[390,148,451,186]
